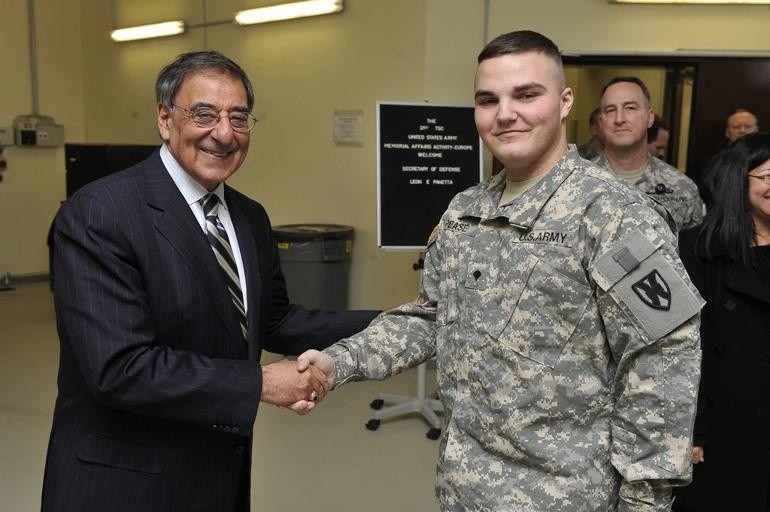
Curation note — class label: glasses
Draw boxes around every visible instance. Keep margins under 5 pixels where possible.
[168,101,259,134]
[747,172,770,185]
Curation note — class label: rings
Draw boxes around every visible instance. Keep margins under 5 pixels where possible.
[310,389,320,403]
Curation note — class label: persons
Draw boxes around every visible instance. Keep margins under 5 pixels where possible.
[672,132,768,508]
[574,108,606,162]
[34,52,384,511]
[276,31,705,512]
[583,79,702,254]
[726,109,760,145]
[645,114,670,161]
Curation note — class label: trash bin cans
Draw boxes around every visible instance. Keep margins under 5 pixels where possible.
[271,224,355,360]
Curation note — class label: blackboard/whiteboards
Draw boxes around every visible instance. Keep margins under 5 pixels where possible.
[376,101,484,250]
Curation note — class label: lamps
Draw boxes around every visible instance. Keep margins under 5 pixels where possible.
[111,19,186,43]
[232,0,345,26]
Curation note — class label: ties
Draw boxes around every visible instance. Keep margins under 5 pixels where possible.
[198,192,250,342]
